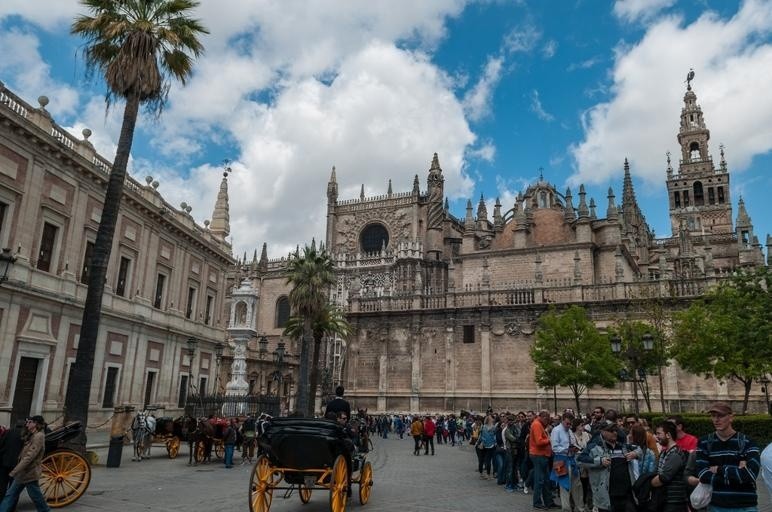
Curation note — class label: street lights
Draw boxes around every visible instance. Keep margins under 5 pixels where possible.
[185,337,198,399]
[608,327,656,421]
[215,342,224,398]
[759,371,772,415]
[273,339,291,406]
[257,332,267,399]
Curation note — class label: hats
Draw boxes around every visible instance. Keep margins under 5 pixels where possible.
[598,420,617,432]
[707,402,734,416]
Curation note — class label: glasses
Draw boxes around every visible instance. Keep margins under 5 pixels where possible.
[579,423,586,427]
[564,422,572,426]
[626,420,638,427]
[592,411,601,414]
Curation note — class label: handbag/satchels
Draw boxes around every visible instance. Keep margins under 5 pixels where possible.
[552,459,568,478]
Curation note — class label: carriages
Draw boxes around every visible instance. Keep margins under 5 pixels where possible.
[0,417,95,508]
[248,407,374,512]
[131,408,237,467]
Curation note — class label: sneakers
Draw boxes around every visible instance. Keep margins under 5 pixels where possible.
[478,472,593,512]
[412,446,435,456]
[223,456,254,471]
[377,434,470,447]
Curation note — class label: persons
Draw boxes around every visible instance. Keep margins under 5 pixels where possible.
[0,415,53,512]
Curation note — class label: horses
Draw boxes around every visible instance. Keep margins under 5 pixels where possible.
[131,410,156,461]
[182,414,215,467]
[351,406,368,453]
